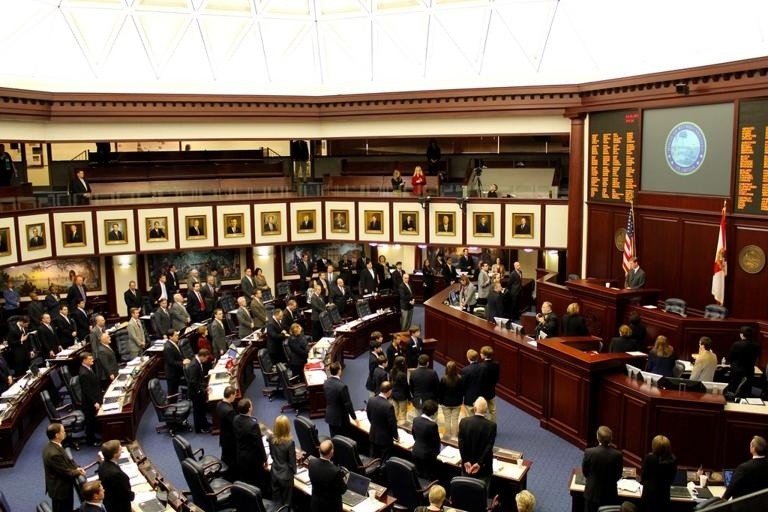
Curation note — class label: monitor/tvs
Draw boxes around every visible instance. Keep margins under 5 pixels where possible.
[449,290,459,307]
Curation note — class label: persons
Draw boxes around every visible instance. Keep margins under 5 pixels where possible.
[427,157,448,178]
[1,276,95,393]
[78,249,473,445]
[391,169,406,192]
[584,426,678,511]
[368,215,451,232]
[291,140,309,182]
[476,216,531,235]
[66,220,164,243]
[45,379,535,512]
[611,313,768,398]
[189,213,347,236]
[29,228,45,246]
[1,144,19,184]
[724,436,767,512]
[411,166,426,194]
[458,263,591,338]
[487,183,498,197]
[73,169,92,204]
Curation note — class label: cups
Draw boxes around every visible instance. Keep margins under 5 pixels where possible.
[517,459,523,469]
[699,474,708,488]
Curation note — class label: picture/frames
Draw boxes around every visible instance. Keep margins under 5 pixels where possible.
[274,245,378,283]
[0,256,115,303]
[137,247,254,296]
[415,246,518,276]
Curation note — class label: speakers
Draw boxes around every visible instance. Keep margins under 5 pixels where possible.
[290,139,308,161]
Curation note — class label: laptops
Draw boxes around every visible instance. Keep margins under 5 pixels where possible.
[341,471,371,507]
[670,468,691,498]
[724,469,734,485]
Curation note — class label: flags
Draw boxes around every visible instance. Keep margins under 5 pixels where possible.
[708,207,729,305]
[621,208,637,274]
